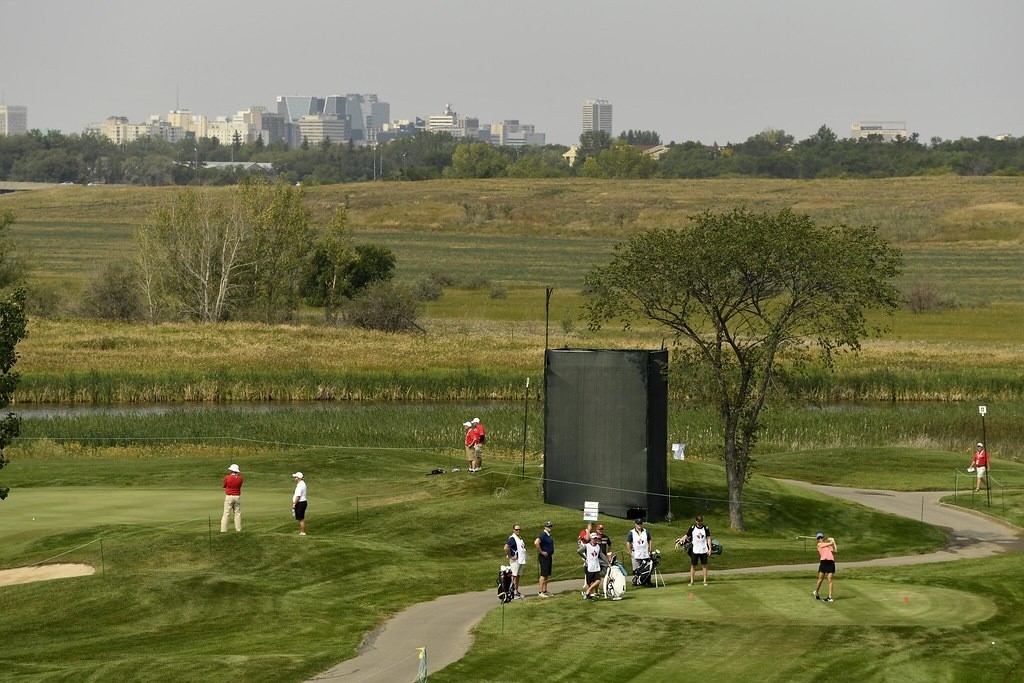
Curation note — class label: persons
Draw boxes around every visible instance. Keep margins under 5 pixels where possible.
[578,532,611,600]
[292,471,308,535]
[470,418,485,471]
[626,519,652,586]
[596,525,612,560]
[220,463,243,533]
[675,515,711,587]
[504,525,527,599]
[578,522,594,548]
[813,533,837,602]
[463,422,477,472]
[969,442,991,492]
[534,521,554,598]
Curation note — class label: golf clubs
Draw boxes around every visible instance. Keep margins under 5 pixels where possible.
[795,535,829,542]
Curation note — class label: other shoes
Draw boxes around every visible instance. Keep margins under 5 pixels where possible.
[467,469,470,473]
[985,487,990,492]
[475,467,482,471]
[974,488,980,492]
[703,581,708,587]
[470,470,474,473]
[298,532,307,536]
[688,582,694,586]
[581,584,622,600]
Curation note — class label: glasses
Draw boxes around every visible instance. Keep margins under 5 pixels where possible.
[514,529,521,531]
[548,525,553,527]
[637,524,642,525]
[294,477,297,479]
[817,537,824,539]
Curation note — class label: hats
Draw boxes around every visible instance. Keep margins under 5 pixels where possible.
[588,522,595,525]
[228,464,240,473]
[596,524,604,530]
[816,533,823,537]
[590,532,598,538]
[292,472,304,479]
[544,521,553,526]
[696,515,703,522]
[977,443,983,447]
[462,421,472,427]
[635,518,642,524]
[471,418,480,424]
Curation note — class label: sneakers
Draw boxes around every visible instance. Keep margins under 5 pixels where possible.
[515,592,525,598]
[511,594,520,600]
[544,591,554,597]
[537,592,548,598]
[812,591,821,600]
[822,598,833,603]
